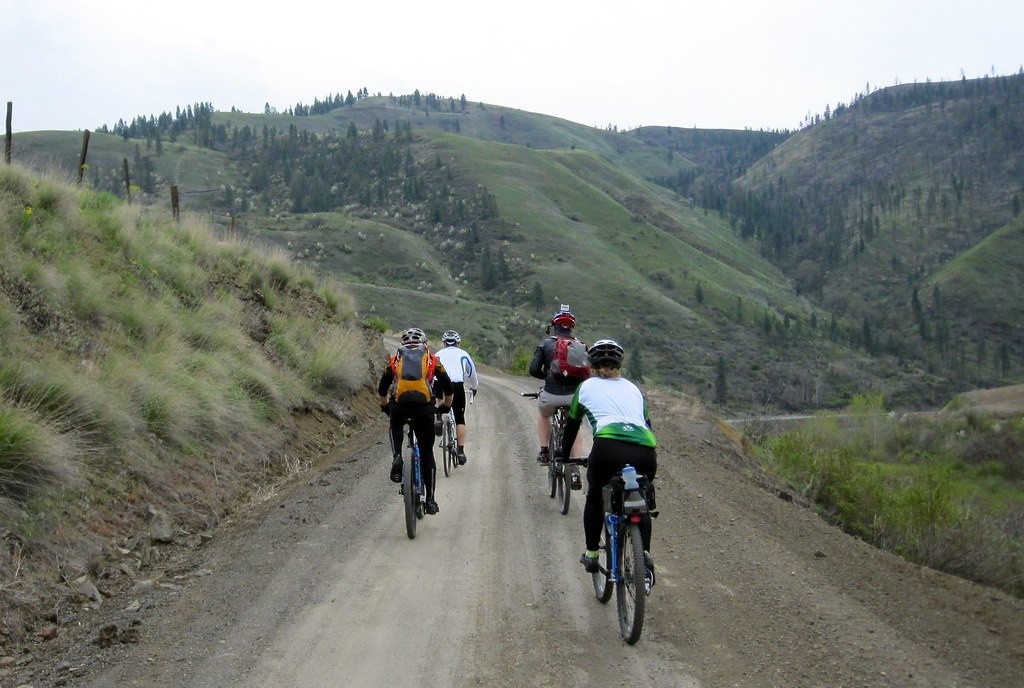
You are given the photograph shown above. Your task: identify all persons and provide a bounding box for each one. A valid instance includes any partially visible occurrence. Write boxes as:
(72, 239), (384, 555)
(555, 340), (659, 586)
(529, 311), (590, 490)
(433, 330), (478, 465)
(378, 328), (453, 515)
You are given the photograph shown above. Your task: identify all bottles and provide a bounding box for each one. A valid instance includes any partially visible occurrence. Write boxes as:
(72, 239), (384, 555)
(605, 511), (612, 535)
(622, 464), (639, 490)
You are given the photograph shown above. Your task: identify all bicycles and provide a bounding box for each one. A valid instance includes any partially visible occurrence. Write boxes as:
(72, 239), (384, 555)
(383, 401), (450, 540)
(430, 388), (474, 477)
(520, 389), (575, 514)
(555, 454), (655, 646)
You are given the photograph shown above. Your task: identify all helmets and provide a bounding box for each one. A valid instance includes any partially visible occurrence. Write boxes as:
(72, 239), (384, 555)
(442, 329), (461, 343)
(552, 311), (577, 327)
(400, 327), (429, 345)
(588, 340), (625, 365)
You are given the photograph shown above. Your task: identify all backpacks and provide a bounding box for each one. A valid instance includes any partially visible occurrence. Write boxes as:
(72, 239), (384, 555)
(550, 334), (590, 381)
(396, 343), (433, 408)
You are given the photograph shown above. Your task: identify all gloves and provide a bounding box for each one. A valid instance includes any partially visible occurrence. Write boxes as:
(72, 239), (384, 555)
(469, 388), (477, 398)
(381, 404), (390, 414)
(437, 404), (452, 415)
(554, 447), (565, 460)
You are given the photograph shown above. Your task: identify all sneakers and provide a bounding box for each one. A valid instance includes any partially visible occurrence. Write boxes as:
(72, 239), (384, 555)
(570, 473), (583, 490)
(537, 452), (551, 463)
(433, 420), (442, 438)
(426, 501), (439, 514)
(580, 552), (599, 574)
(457, 454), (467, 465)
(390, 454), (404, 484)
(641, 555), (655, 585)
